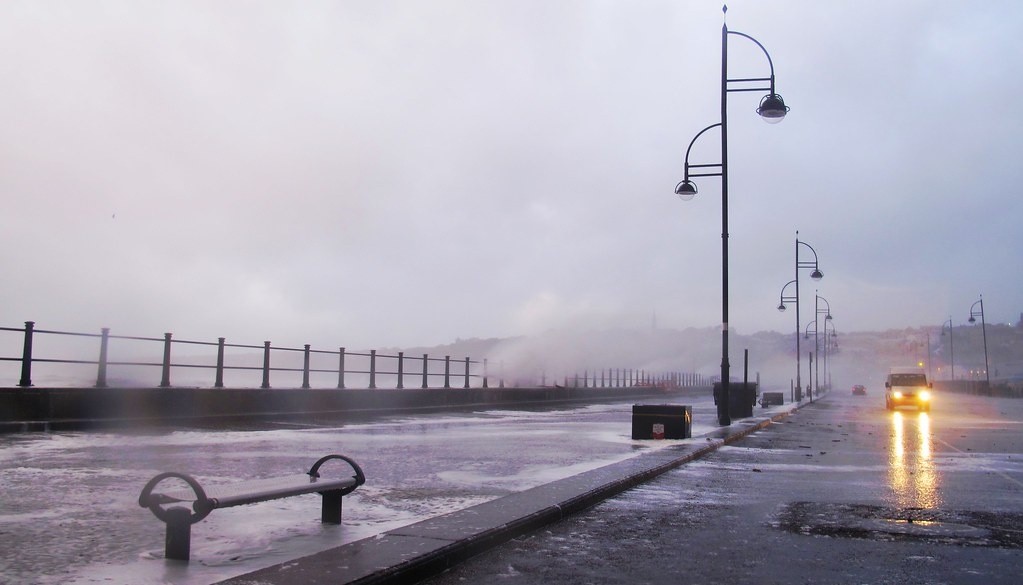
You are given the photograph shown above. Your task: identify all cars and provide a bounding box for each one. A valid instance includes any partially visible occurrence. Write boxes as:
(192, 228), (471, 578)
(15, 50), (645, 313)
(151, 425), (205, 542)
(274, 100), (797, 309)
(852, 384), (866, 395)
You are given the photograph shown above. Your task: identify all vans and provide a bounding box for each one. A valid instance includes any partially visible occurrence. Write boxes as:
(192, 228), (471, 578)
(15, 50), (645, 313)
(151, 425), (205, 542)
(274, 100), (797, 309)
(886, 367), (933, 412)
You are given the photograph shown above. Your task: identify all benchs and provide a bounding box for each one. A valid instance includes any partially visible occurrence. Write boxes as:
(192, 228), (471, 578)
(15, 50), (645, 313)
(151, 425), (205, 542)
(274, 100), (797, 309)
(138, 453), (365, 561)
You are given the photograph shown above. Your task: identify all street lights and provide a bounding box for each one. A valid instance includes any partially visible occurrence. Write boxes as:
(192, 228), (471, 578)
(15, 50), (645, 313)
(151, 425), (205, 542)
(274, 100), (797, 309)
(819, 315), (838, 393)
(804, 289), (834, 397)
(776, 229), (824, 403)
(673, 3), (790, 428)
(940, 315), (955, 393)
(968, 294), (991, 397)
(909, 333), (932, 388)
